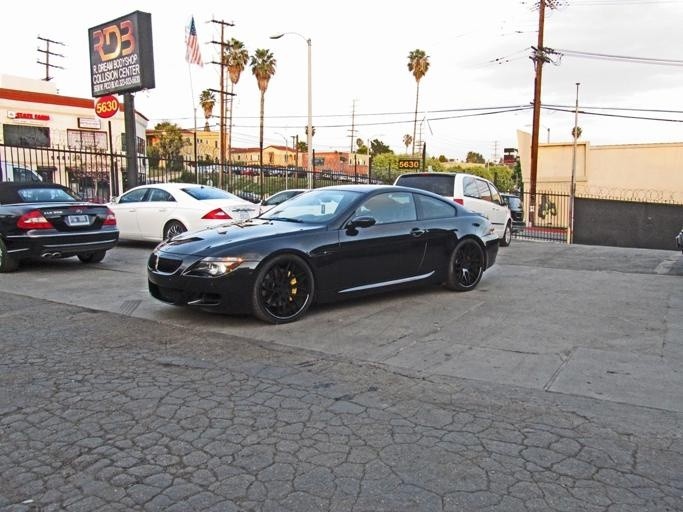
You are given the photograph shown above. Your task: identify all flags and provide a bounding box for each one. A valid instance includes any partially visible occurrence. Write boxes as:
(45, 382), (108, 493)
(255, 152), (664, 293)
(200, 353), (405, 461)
(185, 17), (205, 68)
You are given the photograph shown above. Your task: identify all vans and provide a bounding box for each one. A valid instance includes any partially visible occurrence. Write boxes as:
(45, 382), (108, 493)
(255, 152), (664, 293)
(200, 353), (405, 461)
(392, 171), (516, 248)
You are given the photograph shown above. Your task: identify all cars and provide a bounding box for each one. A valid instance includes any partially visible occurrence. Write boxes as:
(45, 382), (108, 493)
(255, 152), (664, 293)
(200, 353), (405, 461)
(146, 182), (502, 325)
(0, 180), (120, 274)
(93, 180), (261, 247)
(248, 184), (315, 218)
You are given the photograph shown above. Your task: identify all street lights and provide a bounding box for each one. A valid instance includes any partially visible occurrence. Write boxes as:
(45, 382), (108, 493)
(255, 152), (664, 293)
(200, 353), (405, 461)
(267, 28), (320, 191)
